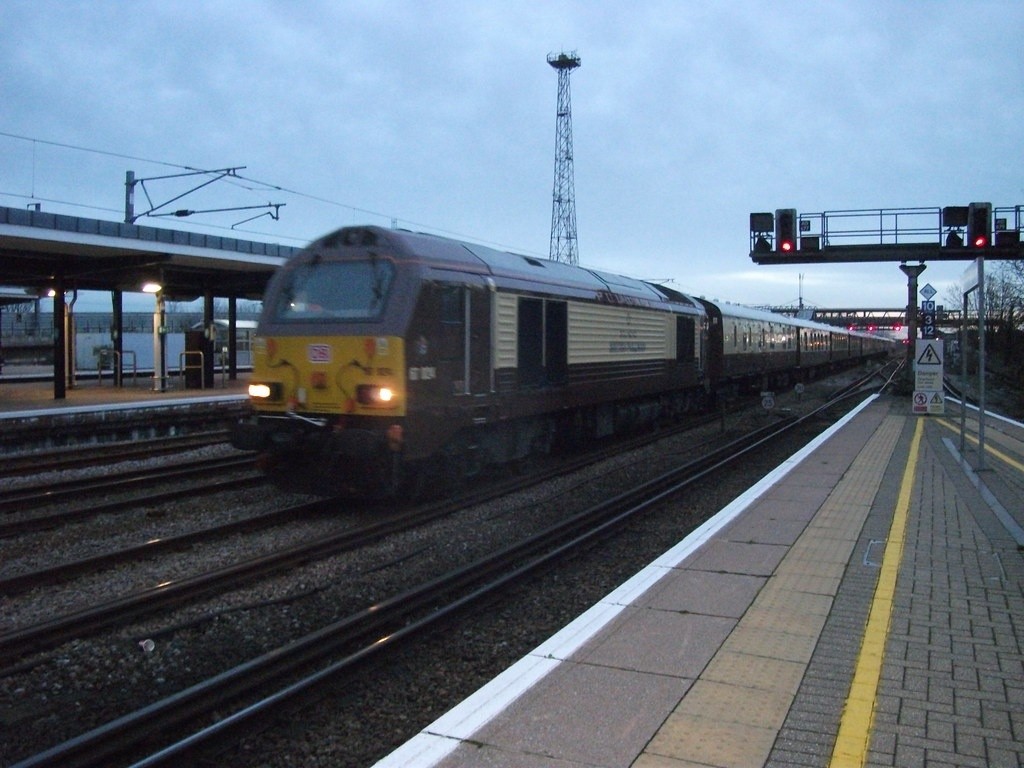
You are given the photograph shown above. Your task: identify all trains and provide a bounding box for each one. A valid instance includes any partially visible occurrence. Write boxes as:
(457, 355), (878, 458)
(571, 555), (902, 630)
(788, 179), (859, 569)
(230, 222), (901, 487)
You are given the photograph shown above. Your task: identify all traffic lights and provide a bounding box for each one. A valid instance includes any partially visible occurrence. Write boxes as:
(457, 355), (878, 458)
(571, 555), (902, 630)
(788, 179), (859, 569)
(775, 208), (797, 252)
(969, 203), (991, 248)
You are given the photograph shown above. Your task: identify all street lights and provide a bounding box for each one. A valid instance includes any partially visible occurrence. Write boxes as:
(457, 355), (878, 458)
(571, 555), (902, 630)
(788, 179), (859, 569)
(140, 282), (164, 390)
(47, 289), (75, 388)
